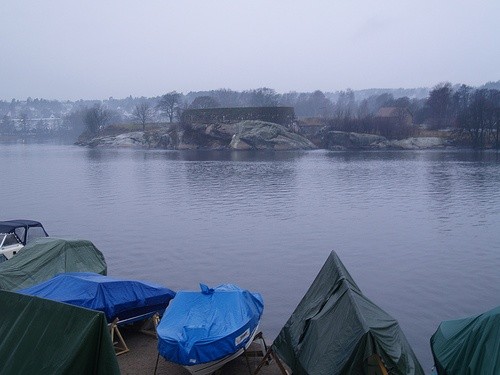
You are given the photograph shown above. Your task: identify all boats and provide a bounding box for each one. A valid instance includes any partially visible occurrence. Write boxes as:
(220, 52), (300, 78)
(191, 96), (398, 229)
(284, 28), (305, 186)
(157, 283), (265, 375)
